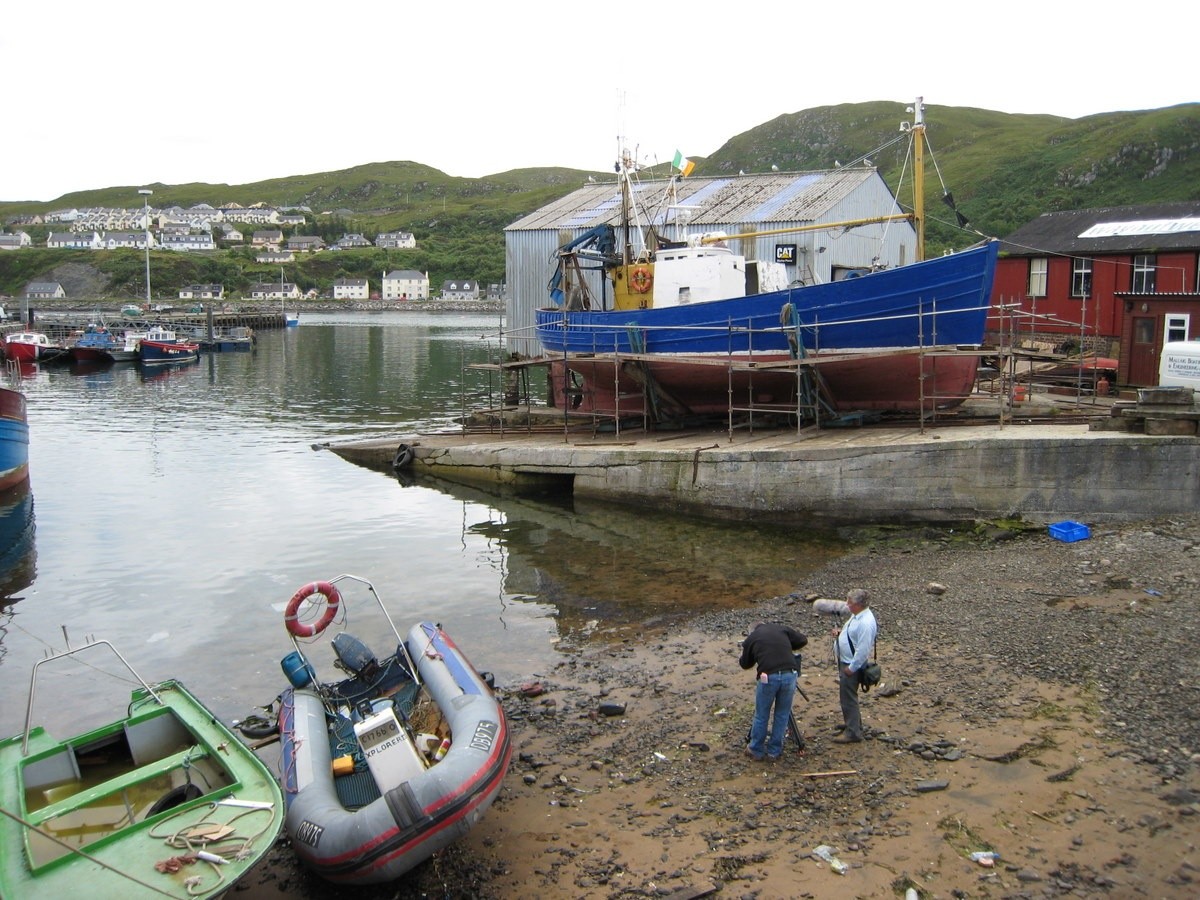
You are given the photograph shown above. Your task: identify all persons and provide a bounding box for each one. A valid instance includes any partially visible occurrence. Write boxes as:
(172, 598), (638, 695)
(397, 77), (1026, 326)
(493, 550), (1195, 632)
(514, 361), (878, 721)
(830, 588), (878, 744)
(738, 620), (808, 762)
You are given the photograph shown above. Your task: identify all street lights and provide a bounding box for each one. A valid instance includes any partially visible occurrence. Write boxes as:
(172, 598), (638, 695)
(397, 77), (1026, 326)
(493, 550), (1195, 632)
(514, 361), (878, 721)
(138, 189), (154, 312)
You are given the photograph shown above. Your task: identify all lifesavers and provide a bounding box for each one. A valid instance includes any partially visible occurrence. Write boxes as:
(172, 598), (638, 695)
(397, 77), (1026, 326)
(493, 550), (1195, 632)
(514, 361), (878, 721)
(284, 581), (339, 637)
(630, 266), (652, 294)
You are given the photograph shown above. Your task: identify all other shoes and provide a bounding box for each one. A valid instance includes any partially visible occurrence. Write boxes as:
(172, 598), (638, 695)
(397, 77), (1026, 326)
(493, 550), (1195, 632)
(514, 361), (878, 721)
(833, 733), (860, 743)
(767, 754), (777, 762)
(835, 723), (845, 729)
(743, 748), (763, 761)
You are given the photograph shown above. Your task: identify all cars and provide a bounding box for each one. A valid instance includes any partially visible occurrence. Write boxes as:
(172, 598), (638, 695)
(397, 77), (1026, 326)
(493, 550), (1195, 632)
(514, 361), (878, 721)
(120, 305), (144, 317)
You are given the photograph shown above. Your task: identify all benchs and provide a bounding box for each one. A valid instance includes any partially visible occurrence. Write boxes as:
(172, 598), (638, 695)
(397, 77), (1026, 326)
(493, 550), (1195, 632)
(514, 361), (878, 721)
(28, 744), (209, 831)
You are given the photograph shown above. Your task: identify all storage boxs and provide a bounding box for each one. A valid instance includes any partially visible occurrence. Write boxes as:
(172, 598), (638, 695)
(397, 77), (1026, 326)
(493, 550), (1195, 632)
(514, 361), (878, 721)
(1048, 520), (1090, 543)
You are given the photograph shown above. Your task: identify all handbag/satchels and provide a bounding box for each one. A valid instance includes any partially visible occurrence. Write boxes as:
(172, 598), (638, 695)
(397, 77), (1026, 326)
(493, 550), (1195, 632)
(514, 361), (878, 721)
(856, 664), (881, 692)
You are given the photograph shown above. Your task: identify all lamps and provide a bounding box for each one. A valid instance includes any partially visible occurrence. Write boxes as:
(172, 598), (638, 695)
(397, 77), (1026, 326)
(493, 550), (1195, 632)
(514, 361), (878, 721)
(1141, 303), (1148, 313)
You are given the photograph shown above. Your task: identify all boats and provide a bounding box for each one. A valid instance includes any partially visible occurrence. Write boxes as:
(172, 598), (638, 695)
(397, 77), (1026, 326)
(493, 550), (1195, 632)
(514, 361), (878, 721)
(138, 340), (200, 368)
(278, 572), (512, 888)
(0, 388), (31, 492)
(0, 625), (285, 900)
(0, 477), (37, 601)
(532, 95), (1001, 421)
(69, 332), (137, 365)
(241, 716), (278, 743)
(5, 331), (69, 364)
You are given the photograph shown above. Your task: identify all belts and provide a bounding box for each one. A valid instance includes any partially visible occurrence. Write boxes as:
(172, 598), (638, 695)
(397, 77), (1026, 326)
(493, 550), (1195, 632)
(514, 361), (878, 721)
(777, 669), (796, 673)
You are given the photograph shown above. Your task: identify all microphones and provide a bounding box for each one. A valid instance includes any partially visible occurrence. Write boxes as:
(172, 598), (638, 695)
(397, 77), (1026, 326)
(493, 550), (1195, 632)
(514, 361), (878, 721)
(812, 599), (850, 616)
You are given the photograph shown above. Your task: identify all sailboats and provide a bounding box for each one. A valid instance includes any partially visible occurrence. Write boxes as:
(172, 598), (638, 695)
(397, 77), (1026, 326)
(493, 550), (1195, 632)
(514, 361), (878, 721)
(280, 267), (299, 327)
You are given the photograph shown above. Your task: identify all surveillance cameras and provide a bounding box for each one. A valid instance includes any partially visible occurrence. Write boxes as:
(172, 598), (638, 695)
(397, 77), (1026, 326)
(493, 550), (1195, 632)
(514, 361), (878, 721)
(905, 109), (910, 114)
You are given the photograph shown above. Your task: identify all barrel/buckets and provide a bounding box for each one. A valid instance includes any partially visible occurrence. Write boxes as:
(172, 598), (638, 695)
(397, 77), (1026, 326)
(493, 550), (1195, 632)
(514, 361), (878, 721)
(280, 652), (315, 688)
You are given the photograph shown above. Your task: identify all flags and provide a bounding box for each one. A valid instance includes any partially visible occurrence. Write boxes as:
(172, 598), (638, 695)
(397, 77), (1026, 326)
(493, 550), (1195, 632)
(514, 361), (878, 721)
(671, 149), (695, 178)
